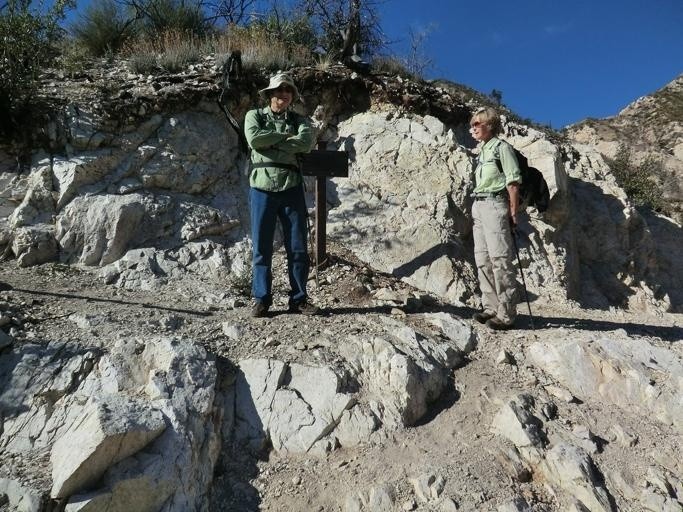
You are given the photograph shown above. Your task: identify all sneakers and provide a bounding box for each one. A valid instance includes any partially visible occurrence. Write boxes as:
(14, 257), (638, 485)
(472, 312), (495, 324)
(290, 302), (321, 315)
(486, 317), (513, 329)
(251, 303), (269, 317)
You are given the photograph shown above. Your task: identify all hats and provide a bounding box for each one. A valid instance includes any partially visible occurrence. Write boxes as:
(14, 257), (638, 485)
(259, 73), (300, 104)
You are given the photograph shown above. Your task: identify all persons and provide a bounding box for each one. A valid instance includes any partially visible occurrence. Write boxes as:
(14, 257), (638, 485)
(470, 108), (522, 333)
(243, 72), (319, 317)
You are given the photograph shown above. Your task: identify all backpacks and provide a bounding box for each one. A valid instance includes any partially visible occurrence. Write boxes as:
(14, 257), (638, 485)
(494, 139), (530, 206)
